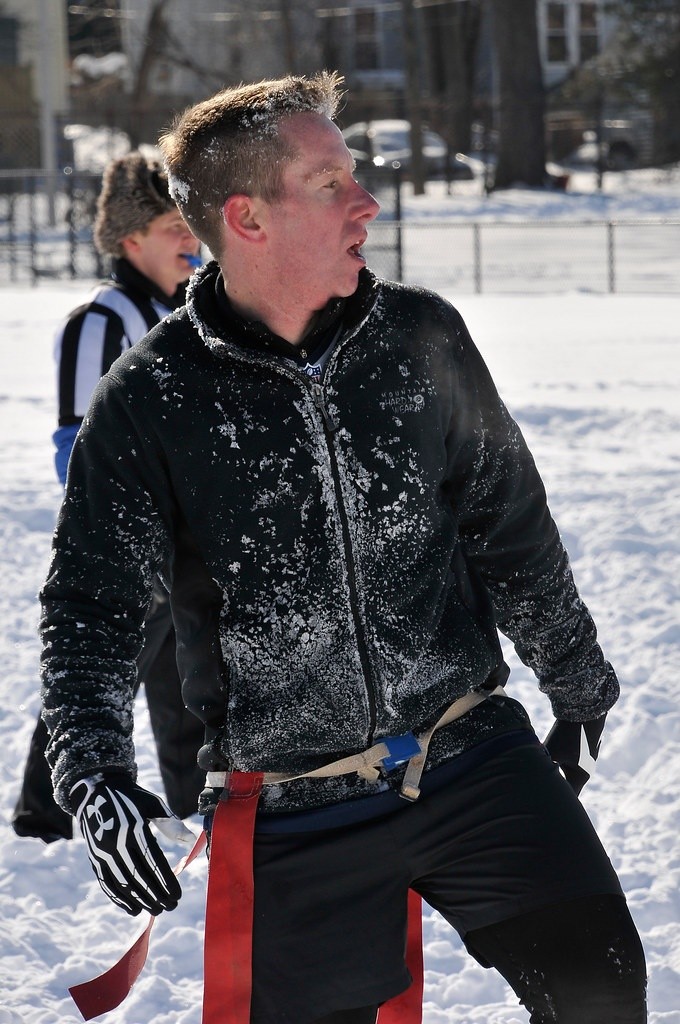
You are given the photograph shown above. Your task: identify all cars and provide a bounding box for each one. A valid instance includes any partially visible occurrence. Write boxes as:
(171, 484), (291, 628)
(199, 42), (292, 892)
(339, 117), (475, 185)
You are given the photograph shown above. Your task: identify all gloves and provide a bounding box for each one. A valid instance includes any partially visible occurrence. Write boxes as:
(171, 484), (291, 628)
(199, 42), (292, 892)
(545, 713), (607, 800)
(69, 764), (198, 917)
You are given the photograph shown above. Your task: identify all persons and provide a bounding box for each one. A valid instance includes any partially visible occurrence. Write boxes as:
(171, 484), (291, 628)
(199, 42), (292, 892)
(39, 71), (650, 1024)
(50, 154), (206, 820)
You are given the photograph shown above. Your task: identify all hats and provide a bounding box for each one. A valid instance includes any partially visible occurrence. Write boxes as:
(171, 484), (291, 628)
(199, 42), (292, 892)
(92, 155), (178, 253)
